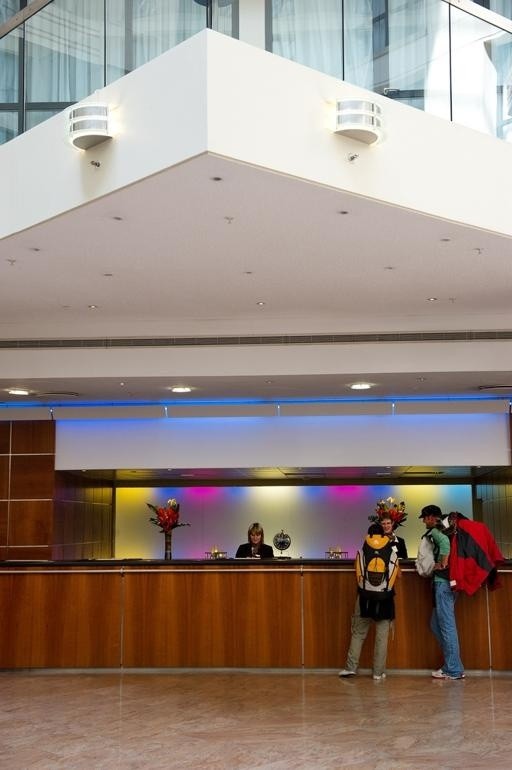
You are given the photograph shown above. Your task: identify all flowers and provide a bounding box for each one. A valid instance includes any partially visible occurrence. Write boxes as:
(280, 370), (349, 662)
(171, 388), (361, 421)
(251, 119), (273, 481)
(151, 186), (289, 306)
(146, 498), (193, 534)
(367, 495), (408, 533)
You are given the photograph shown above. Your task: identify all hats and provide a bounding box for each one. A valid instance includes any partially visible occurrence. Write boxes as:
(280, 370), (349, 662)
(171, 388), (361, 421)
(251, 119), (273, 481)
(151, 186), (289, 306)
(418, 505), (441, 518)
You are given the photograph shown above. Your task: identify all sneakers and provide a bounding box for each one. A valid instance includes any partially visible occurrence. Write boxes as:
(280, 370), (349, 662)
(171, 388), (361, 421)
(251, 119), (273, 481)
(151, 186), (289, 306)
(432, 670), (465, 680)
(373, 674), (386, 679)
(338, 669), (356, 678)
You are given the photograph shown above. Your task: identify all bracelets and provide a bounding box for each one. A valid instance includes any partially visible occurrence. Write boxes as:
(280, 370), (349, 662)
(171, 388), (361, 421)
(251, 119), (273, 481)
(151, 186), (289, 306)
(441, 564), (449, 570)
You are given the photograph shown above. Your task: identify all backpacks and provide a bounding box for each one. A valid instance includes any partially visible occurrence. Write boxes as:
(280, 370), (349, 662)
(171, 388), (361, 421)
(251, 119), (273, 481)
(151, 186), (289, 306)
(415, 526), (440, 577)
(360, 540), (394, 593)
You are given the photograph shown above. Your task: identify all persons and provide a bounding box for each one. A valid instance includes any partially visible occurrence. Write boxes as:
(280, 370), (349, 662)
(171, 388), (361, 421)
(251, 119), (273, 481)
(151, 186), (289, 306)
(234, 522), (275, 558)
(378, 512), (408, 559)
(415, 504), (467, 681)
(338, 524), (401, 682)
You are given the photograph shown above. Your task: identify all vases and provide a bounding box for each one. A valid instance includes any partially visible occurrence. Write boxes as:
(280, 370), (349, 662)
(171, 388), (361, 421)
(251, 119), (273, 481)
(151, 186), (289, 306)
(164, 532), (172, 561)
